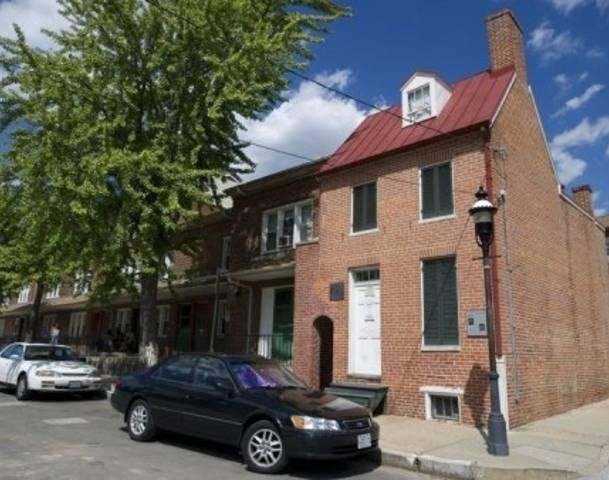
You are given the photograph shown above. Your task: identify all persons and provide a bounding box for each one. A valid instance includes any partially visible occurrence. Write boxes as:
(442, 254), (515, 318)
(96, 323), (136, 352)
(50, 323), (60, 344)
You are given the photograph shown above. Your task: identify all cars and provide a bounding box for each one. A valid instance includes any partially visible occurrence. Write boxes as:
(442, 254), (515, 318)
(106, 349), (381, 475)
(0, 341), (103, 399)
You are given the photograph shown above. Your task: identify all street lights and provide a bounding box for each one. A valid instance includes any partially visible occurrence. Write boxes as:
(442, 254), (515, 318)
(463, 186), (511, 457)
